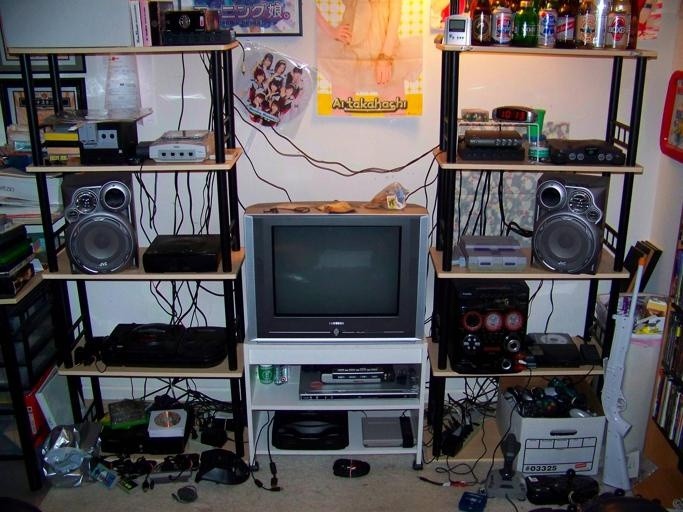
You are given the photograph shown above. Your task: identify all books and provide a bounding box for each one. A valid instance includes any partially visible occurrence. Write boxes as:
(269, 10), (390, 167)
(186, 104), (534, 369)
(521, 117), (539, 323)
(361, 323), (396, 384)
(35, 363), (74, 432)
(650, 236), (683, 454)
(0, 222), (36, 299)
(43, 125), (81, 161)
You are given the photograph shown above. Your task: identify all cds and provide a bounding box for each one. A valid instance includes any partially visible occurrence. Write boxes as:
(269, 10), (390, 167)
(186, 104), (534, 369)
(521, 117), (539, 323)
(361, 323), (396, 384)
(541, 334), (568, 347)
(154, 410), (181, 429)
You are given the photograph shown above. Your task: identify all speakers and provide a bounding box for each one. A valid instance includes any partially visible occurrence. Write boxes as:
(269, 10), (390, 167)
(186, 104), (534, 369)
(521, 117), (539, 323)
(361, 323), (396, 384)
(447, 278), (529, 374)
(60, 172), (139, 273)
(531, 173), (608, 275)
(444, 15), (472, 47)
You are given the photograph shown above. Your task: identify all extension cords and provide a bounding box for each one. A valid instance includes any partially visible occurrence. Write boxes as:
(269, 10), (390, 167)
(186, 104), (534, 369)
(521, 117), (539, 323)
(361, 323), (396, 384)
(445, 416), (481, 456)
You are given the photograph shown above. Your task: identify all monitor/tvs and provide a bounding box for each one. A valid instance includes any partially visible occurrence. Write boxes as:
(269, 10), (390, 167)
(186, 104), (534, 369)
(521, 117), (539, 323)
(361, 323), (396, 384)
(242, 202), (428, 344)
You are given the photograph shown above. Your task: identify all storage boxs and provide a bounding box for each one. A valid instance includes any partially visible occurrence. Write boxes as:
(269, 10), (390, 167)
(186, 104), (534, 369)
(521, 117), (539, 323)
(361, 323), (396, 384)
(492, 376), (607, 478)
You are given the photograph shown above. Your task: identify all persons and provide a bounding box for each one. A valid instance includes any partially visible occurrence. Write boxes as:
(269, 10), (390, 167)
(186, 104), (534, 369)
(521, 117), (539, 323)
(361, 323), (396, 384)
(319, 0), (405, 116)
(242, 53), (303, 129)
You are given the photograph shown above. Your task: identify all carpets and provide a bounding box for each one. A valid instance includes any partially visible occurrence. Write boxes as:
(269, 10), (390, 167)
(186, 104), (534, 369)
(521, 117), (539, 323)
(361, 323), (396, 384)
(34, 408), (638, 512)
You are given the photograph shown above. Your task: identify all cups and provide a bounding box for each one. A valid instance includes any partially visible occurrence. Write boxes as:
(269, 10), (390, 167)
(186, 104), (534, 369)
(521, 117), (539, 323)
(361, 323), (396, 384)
(528, 110), (545, 136)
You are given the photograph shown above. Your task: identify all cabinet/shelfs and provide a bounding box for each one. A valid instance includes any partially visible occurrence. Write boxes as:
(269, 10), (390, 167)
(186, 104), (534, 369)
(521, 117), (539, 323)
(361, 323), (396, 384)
(649, 204), (683, 472)
(239, 343), (431, 468)
(426, 32), (655, 468)
(10, 38), (248, 462)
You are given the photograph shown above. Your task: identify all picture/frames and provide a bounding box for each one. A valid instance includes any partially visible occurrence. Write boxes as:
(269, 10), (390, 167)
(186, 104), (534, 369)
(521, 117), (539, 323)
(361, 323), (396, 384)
(1, 76), (89, 147)
(0, 27), (88, 73)
(658, 68), (682, 165)
(175, 0), (303, 36)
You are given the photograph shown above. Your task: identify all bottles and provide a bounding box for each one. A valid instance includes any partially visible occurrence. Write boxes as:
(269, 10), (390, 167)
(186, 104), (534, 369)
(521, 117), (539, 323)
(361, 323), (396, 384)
(576, 0), (594, 49)
(605, 0), (631, 50)
(557, 1), (577, 48)
(628, 0), (637, 50)
(522, 134), (549, 164)
(257, 365), (287, 385)
(513, 1), (539, 46)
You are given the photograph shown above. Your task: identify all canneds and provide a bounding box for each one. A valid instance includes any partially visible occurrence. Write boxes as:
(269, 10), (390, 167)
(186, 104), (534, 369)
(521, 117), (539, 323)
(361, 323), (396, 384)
(605, 11), (632, 50)
(537, 6), (556, 48)
(492, 6), (514, 47)
(258, 364), (286, 386)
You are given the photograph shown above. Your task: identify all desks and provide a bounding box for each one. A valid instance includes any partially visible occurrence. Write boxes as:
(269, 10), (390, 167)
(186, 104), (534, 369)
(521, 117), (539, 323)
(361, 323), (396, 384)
(1, 257), (87, 496)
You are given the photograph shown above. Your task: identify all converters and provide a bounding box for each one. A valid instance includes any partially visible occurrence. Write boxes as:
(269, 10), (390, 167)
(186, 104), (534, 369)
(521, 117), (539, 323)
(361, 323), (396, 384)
(202, 429), (226, 448)
(444, 434), (462, 455)
